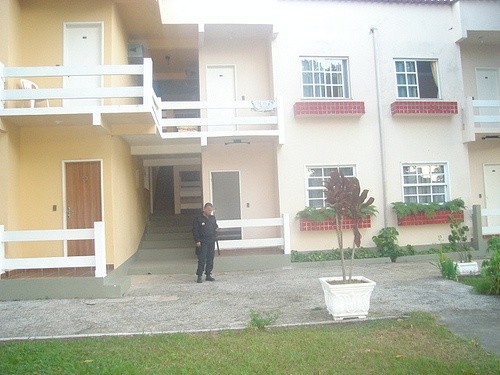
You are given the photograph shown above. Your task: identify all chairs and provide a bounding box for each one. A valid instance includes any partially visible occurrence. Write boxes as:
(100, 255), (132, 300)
(20, 79), (48, 108)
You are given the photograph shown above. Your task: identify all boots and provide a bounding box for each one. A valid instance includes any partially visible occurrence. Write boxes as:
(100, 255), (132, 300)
(205, 273), (215, 281)
(197, 275), (202, 282)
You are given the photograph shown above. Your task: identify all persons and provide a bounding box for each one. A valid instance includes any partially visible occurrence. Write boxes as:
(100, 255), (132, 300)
(192, 203), (219, 283)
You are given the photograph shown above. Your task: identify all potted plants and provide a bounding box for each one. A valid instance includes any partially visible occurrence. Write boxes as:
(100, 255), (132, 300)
(319, 169), (376, 322)
(449, 212), (478, 276)
